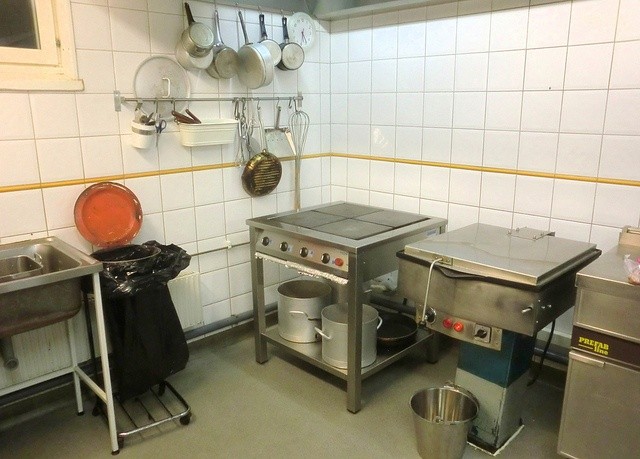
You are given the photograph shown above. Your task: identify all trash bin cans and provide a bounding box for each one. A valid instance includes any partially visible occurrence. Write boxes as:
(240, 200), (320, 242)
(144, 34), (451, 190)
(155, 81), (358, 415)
(77, 180), (193, 399)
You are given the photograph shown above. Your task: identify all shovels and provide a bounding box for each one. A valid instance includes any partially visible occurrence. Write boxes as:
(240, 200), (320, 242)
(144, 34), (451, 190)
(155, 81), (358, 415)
(265, 105), (297, 159)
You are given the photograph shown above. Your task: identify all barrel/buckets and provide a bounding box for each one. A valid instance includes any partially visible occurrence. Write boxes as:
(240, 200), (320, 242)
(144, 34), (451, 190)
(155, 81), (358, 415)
(409, 381), (481, 459)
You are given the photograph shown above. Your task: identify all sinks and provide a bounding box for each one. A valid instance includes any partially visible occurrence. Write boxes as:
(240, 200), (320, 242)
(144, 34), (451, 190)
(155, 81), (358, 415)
(0, 234), (103, 339)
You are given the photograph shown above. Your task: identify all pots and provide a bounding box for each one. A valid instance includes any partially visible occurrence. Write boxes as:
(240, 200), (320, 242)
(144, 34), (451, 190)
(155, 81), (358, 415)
(182, 2), (214, 56)
(278, 15), (306, 71)
(176, 22), (214, 72)
(259, 12), (283, 68)
(207, 8), (238, 79)
(242, 117), (281, 198)
(374, 313), (418, 348)
(238, 11), (274, 91)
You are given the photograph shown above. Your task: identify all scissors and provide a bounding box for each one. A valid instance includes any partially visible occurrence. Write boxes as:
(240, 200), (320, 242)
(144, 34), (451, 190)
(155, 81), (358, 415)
(154, 119), (168, 147)
